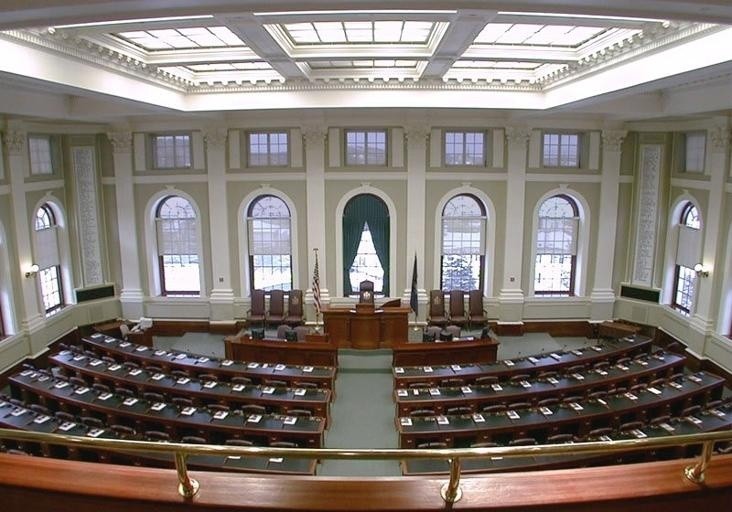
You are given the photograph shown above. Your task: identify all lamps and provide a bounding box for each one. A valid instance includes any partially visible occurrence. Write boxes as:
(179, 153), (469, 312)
(694, 262), (711, 278)
(26, 261), (39, 279)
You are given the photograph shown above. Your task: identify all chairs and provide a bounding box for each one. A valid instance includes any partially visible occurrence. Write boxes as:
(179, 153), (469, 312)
(426, 289), (489, 330)
(236, 288), (305, 328)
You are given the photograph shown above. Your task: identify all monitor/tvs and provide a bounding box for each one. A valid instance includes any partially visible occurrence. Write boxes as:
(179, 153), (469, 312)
(480, 327), (489, 340)
(285, 330), (297, 341)
(252, 328), (265, 339)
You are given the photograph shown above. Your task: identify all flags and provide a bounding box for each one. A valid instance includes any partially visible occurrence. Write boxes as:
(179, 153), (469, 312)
(409, 255), (420, 317)
(311, 248), (323, 316)
